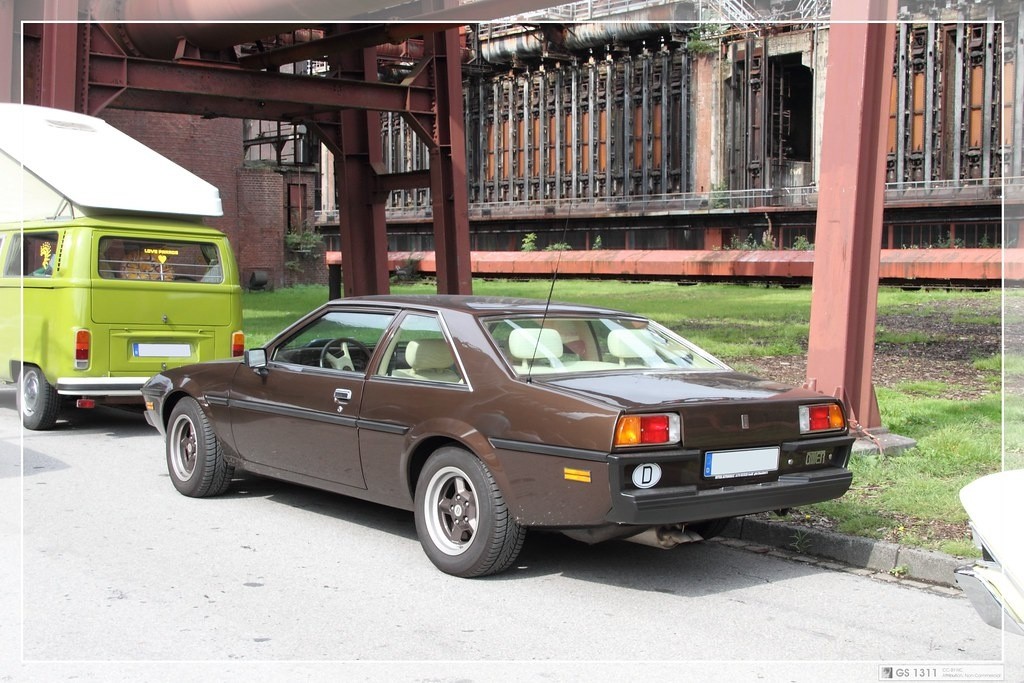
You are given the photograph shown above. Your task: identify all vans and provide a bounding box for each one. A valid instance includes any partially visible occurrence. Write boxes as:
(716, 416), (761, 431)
(0, 213), (246, 430)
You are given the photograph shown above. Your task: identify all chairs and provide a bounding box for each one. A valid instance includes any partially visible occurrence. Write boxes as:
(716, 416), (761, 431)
(391, 338), (460, 383)
(509, 328), (564, 374)
(608, 329), (655, 368)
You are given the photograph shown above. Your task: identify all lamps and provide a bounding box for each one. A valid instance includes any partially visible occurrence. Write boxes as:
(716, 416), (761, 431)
(244, 270), (268, 292)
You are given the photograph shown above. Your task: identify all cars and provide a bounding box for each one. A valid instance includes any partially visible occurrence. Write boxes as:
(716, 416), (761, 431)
(237, 434), (246, 437)
(144, 297), (853, 580)
